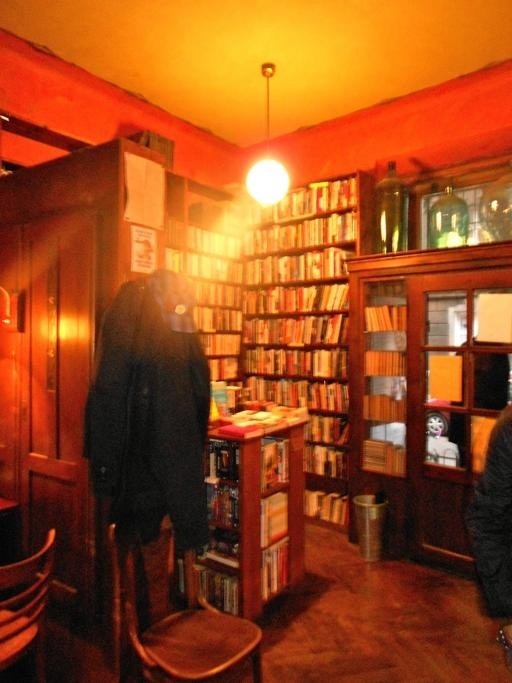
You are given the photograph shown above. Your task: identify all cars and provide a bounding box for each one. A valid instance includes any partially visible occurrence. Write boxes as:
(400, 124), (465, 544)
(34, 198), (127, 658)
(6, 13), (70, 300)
(426, 399), (452, 434)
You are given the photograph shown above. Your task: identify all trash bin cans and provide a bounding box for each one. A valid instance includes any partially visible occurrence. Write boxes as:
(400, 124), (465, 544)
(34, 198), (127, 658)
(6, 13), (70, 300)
(352, 494), (390, 562)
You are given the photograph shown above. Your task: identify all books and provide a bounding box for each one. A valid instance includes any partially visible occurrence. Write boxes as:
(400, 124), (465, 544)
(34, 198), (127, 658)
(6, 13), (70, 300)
(167, 175), (359, 616)
(363, 300), (406, 477)
